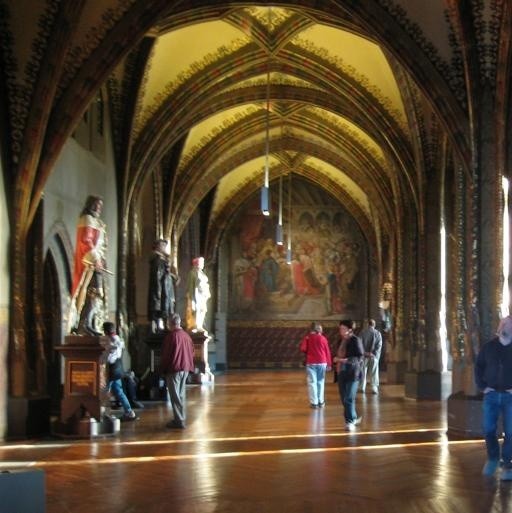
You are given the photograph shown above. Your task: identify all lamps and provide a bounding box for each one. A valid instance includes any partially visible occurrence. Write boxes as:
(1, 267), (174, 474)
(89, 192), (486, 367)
(260, 7), (295, 265)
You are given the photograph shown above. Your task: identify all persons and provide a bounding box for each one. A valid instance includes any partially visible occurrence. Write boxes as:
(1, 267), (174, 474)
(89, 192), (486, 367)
(291, 254), (319, 295)
(298, 319), (333, 409)
(121, 374), (146, 408)
(357, 318), (385, 394)
(257, 247), (281, 292)
(332, 261), (355, 315)
(184, 256), (211, 335)
(147, 237), (179, 334)
(68, 192), (111, 337)
(314, 262), (338, 314)
(99, 320), (137, 422)
(243, 254), (258, 301)
(330, 319), (367, 432)
(157, 312), (195, 430)
(472, 314), (511, 483)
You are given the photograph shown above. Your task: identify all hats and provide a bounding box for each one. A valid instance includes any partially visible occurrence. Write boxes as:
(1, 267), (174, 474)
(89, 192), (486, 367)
(498, 317), (512, 346)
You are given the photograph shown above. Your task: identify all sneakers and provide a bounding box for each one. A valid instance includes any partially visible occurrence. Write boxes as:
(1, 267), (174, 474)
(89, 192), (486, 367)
(311, 404), (319, 410)
(501, 469), (512, 481)
(482, 458), (498, 476)
(320, 402), (326, 408)
(166, 419), (185, 429)
(360, 386), (379, 394)
(344, 419), (357, 431)
(118, 412), (136, 422)
